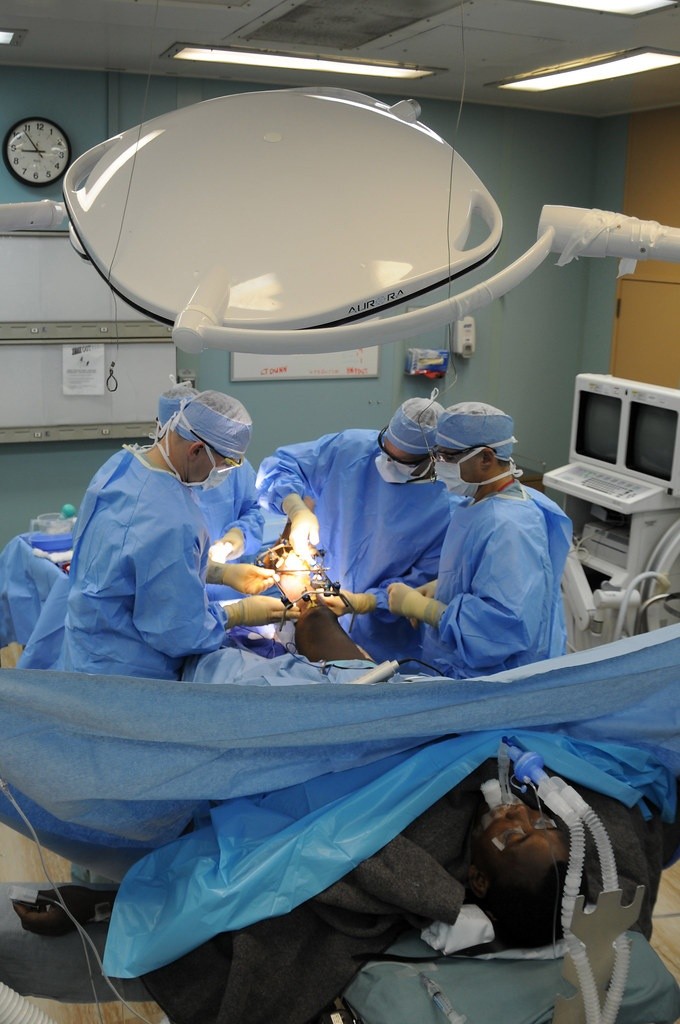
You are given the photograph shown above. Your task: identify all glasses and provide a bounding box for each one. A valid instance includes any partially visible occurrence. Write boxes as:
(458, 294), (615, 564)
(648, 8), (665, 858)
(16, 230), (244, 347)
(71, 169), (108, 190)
(376, 426), (429, 466)
(189, 429), (244, 474)
(430, 442), (497, 464)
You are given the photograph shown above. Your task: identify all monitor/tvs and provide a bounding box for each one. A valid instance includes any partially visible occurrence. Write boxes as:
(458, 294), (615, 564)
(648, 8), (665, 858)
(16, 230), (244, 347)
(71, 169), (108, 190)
(569, 375), (680, 496)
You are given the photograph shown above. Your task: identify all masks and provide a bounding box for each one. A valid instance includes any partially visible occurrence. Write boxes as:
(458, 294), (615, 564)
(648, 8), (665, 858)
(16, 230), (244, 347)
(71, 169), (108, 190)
(189, 445), (231, 492)
(374, 451), (433, 483)
(434, 447), (487, 497)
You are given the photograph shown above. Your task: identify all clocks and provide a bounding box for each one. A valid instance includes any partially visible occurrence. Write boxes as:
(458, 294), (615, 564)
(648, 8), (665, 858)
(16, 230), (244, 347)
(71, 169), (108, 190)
(2, 116), (72, 187)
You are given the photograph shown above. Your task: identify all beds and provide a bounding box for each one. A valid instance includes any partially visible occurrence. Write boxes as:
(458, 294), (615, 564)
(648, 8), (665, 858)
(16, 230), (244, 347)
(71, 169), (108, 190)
(341, 923), (680, 1024)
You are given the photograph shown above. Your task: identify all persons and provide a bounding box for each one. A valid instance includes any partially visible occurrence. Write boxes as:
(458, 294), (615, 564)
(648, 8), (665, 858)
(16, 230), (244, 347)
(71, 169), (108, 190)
(386, 403), (573, 679)
(61, 389), (301, 680)
(255, 396), (451, 674)
(278, 549), (588, 948)
(156, 378), (266, 601)
